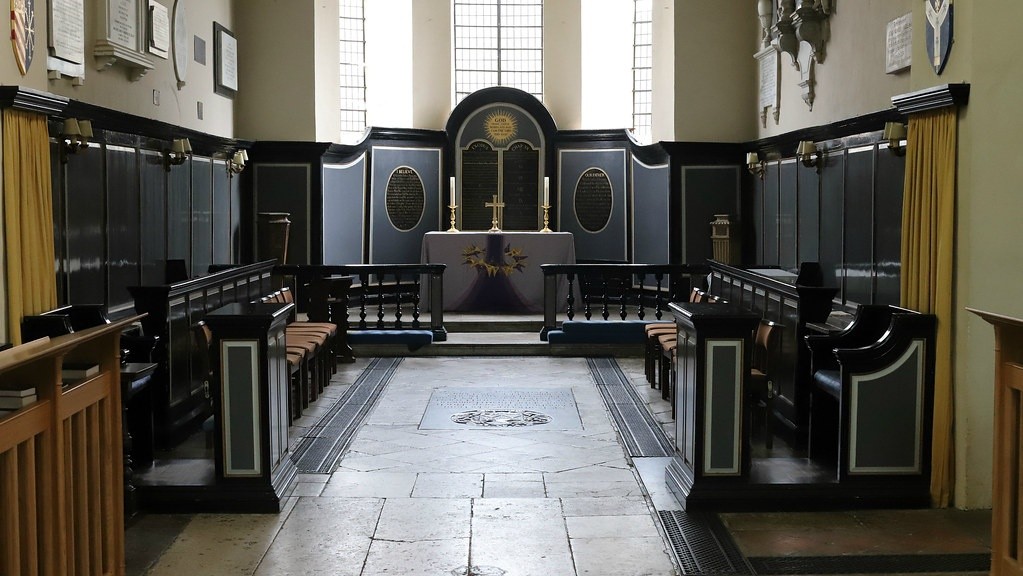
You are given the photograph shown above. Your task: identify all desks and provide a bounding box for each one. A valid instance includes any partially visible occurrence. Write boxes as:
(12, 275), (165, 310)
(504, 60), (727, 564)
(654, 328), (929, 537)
(419, 231), (582, 313)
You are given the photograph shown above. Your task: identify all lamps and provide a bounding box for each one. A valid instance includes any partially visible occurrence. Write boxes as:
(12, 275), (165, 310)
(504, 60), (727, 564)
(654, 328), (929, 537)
(797, 141), (823, 175)
(163, 137), (192, 172)
(883, 120), (907, 149)
(747, 152), (764, 179)
(59, 118), (94, 163)
(230, 148), (249, 178)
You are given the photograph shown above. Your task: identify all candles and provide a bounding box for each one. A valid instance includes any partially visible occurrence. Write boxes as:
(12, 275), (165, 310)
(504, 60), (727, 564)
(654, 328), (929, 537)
(544, 177), (549, 207)
(450, 177), (455, 206)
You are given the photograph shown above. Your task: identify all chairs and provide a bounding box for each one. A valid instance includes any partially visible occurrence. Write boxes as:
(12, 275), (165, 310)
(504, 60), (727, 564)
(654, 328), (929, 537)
(645, 286), (786, 451)
(191, 287), (338, 427)
